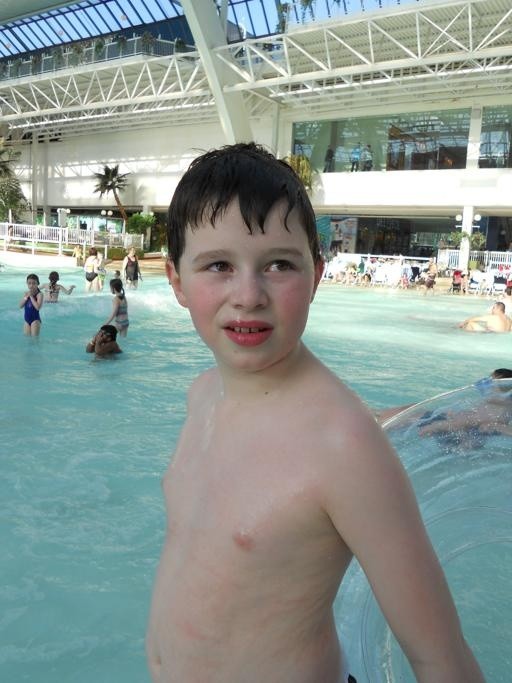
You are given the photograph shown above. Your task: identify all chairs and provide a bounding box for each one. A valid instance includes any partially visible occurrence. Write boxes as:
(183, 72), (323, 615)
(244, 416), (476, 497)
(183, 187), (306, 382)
(327, 258), (507, 296)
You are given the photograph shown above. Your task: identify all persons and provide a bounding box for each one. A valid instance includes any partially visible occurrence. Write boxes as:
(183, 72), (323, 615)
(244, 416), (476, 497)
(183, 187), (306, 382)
(19, 242), (142, 359)
(143, 139), (486, 683)
(377, 370), (512, 454)
(349, 141), (373, 172)
(341, 253), (512, 332)
(323, 145), (334, 172)
